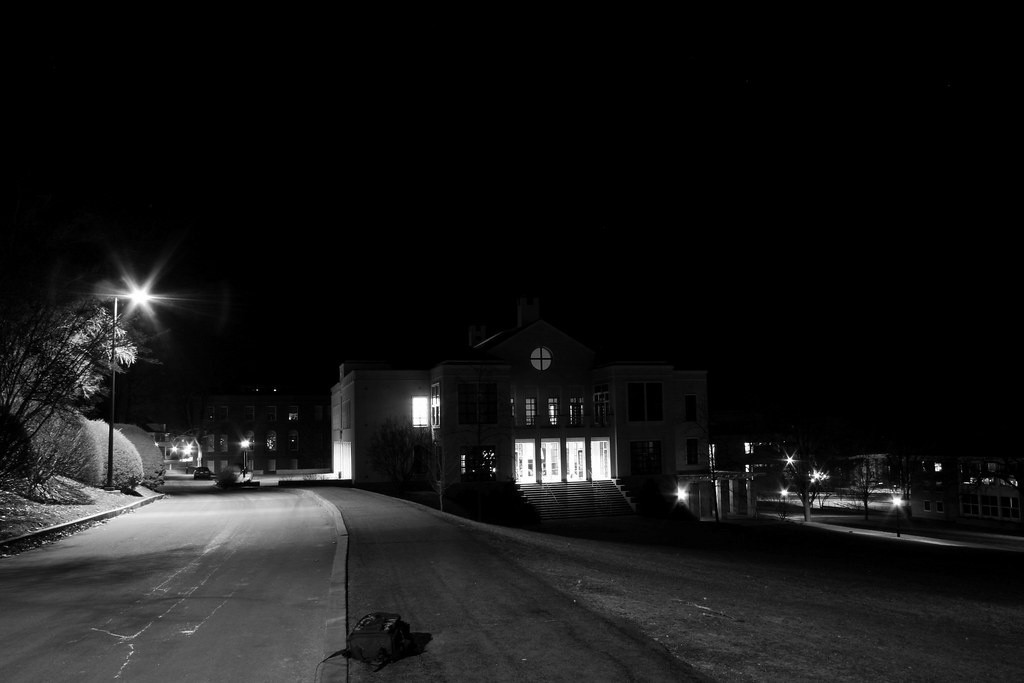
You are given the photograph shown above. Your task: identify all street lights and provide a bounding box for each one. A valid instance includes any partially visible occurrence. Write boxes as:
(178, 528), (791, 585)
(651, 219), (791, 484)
(243, 441), (250, 478)
(104, 289), (150, 491)
(893, 497), (902, 537)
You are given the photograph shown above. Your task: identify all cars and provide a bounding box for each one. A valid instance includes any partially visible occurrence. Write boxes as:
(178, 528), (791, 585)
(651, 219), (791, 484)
(193, 466), (212, 480)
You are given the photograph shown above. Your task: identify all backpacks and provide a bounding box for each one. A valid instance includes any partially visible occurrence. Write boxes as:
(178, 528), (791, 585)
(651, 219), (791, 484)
(342, 611), (411, 672)
